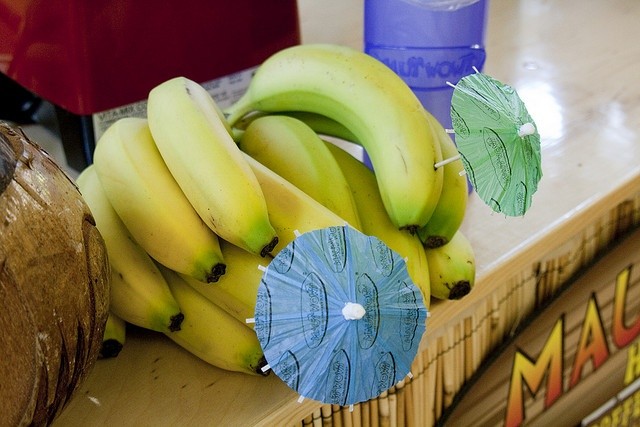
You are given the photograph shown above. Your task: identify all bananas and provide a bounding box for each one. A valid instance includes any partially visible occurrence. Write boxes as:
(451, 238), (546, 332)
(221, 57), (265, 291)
(76, 45), (475, 376)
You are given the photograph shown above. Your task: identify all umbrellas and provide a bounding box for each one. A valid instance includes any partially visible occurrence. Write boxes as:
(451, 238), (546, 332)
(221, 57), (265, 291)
(434, 66), (543, 221)
(246, 221), (431, 412)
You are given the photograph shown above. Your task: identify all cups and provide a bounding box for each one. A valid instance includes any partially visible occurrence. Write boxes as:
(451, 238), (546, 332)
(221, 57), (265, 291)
(364, 1), (485, 194)
